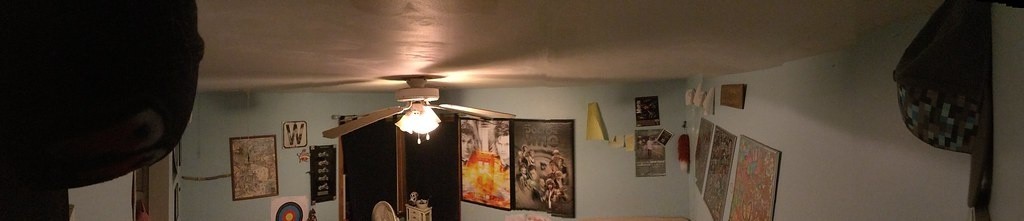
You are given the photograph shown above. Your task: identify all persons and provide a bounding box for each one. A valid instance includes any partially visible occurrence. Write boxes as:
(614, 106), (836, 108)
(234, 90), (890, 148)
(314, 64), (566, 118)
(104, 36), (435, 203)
(518, 146), (567, 209)
(495, 124), (509, 173)
(647, 137), (658, 157)
(461, 121), (475, 168)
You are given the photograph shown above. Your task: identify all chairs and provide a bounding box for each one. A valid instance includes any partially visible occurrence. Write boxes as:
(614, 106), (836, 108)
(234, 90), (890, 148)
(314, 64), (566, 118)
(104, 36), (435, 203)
(372, 200), (399, 221)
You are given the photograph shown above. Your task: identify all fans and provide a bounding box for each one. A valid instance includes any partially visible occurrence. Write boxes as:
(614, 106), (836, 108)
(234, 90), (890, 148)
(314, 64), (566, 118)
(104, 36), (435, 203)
(322, 77), (515, 139)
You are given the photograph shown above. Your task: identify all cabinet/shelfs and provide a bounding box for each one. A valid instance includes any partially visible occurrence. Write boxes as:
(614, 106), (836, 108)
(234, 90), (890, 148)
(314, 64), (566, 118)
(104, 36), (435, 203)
(405, 203), (432, 221)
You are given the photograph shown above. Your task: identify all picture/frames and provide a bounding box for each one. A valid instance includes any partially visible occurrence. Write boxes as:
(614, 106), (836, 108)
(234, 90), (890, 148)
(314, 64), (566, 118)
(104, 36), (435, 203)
(229, 136), (279, 200)
(458, 116), (515, 212)
(514, 118), (575, 217)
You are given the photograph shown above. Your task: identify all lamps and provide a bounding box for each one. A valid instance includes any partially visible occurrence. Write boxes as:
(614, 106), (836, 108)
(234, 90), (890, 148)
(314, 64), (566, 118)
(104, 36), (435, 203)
(395, 100), (441, 144)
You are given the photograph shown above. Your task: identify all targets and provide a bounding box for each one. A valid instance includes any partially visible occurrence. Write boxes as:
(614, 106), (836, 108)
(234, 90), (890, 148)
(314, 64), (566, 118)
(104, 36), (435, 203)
(271, 194), (310, 221)
